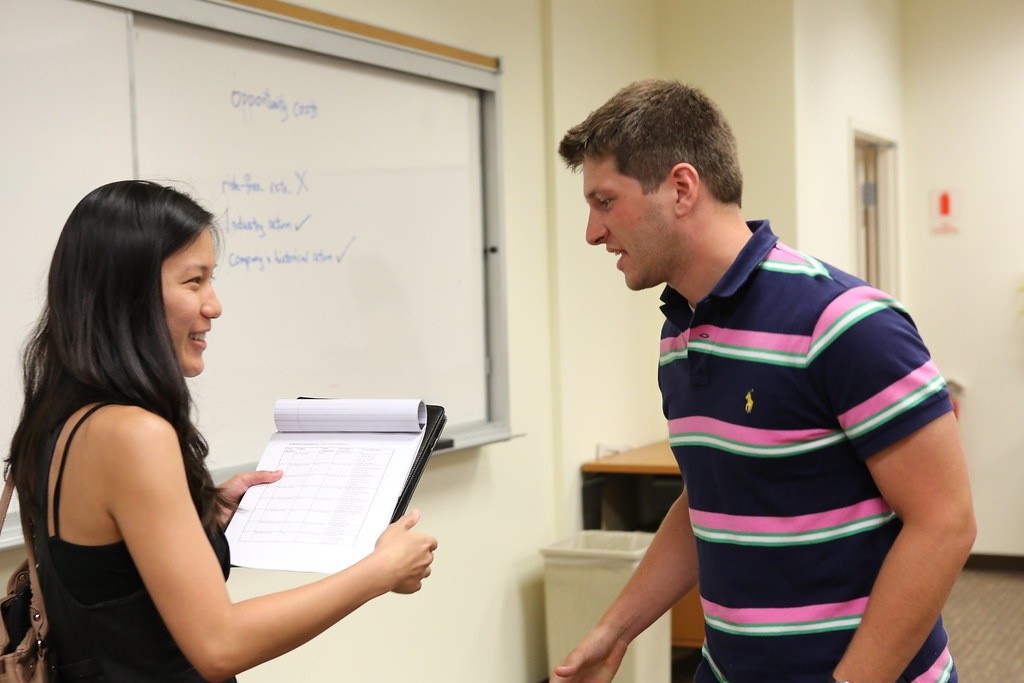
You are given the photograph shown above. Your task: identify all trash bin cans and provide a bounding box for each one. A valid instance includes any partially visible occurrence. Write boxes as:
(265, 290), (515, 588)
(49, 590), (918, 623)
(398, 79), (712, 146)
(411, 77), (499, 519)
(540, 529), (674, 683)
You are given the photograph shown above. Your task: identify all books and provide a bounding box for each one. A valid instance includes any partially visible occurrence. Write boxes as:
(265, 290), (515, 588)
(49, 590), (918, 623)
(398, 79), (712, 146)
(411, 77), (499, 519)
(222, 399), (429, 577)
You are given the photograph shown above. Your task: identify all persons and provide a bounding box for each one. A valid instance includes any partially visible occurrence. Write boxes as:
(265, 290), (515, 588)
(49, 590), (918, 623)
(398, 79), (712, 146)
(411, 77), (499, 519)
(556, 78), (976, 683)
(13, 181), (437, 681)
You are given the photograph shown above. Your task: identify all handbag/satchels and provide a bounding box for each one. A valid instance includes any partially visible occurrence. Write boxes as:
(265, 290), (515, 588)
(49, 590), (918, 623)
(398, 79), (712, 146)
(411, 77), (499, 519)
(0, 556), (54, 683)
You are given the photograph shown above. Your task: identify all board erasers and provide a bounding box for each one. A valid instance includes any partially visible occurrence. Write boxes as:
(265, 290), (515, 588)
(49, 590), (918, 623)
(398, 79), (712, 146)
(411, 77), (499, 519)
(432, 437), (454, 451)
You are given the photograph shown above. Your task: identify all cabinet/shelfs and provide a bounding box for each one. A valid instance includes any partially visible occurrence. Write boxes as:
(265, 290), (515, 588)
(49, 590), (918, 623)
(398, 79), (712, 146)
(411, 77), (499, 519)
(582, 439), (706, 649)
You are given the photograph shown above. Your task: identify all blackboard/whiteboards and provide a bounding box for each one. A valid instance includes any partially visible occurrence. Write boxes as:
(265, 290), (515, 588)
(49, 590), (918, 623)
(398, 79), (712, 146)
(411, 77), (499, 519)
(3, 0), (519, 552)
